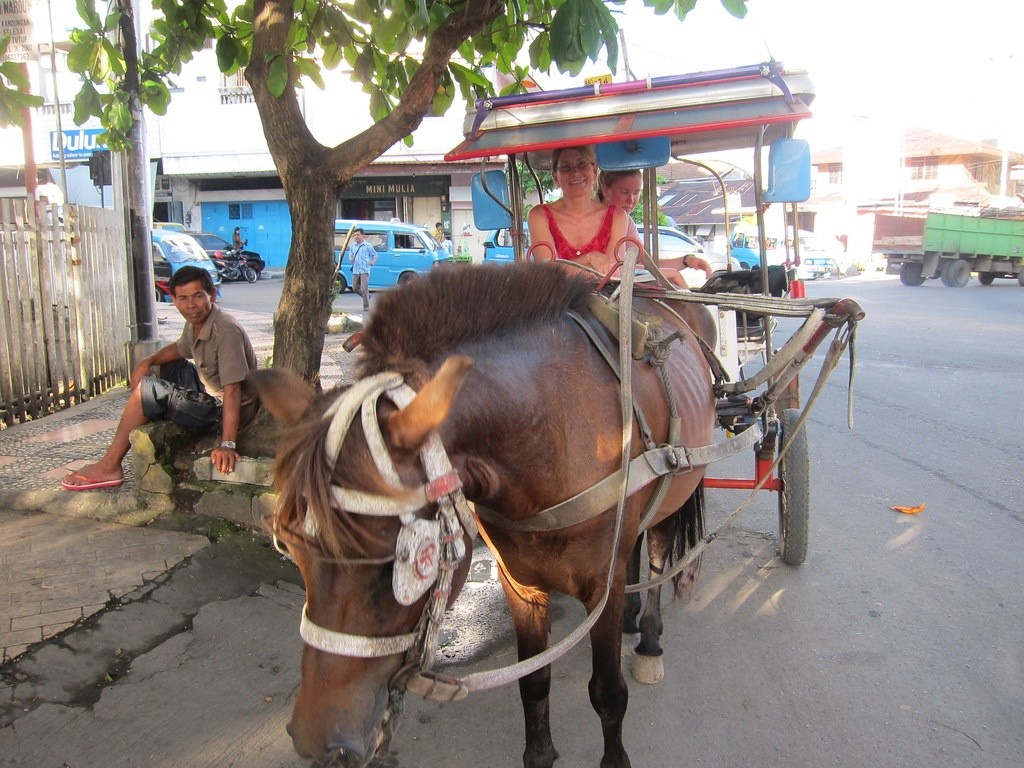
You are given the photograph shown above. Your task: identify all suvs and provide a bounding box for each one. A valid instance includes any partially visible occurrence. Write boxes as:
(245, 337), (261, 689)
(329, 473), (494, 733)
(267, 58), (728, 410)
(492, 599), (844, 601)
(178, 230), (266, 279)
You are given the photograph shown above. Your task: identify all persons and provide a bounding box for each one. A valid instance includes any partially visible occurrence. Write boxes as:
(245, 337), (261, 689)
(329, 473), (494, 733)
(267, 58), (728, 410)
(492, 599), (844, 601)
(349, 228), (378, 311)
(595, 168), (712, 291)
(60, 265), (261, 492)
(233, 227), (242, 249)
(433, 222), (442, 243)
(528, 143), (629, 278)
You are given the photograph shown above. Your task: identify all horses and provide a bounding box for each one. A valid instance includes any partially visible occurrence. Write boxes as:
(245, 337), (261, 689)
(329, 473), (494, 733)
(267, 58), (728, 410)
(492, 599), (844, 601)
(243, 260), (716, 768)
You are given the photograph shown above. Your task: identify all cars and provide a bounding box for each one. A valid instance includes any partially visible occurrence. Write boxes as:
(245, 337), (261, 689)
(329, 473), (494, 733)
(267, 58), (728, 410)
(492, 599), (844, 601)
(635, 224), (744, 289)
(152, 241), (173, 301)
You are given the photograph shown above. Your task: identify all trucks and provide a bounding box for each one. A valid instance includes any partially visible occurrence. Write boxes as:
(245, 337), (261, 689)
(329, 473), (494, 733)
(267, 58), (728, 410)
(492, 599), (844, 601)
(870, 208), (1024, 288)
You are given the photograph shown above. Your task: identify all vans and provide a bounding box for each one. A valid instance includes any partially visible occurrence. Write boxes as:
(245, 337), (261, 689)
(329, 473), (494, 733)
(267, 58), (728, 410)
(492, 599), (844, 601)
(728, 223), (834, 281)
(152, 222), (186, 232)
(152, 229), (223, 303)
(333, 218), (452, 294)
(480, 220), (534, 265)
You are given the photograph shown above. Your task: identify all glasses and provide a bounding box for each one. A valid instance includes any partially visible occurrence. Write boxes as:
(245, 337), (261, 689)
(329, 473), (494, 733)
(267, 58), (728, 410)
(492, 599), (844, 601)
(555, 161), (591, 173)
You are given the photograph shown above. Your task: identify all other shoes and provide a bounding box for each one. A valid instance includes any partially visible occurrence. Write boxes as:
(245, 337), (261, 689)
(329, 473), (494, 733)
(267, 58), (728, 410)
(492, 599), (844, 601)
(364, 306), (369, 311)
(369, 294), (372, 299)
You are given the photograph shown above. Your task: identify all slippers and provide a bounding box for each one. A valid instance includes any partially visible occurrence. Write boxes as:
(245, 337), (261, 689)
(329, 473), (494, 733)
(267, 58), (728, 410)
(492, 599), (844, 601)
(60, 467), (125, 490)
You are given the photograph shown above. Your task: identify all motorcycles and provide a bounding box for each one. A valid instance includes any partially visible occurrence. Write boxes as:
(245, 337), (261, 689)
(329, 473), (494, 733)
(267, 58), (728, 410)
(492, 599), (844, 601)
(211, 238), (258, 283)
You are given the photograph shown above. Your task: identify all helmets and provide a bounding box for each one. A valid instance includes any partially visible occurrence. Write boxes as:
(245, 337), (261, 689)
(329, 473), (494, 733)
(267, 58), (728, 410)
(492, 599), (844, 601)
(213, 252), (224, 260)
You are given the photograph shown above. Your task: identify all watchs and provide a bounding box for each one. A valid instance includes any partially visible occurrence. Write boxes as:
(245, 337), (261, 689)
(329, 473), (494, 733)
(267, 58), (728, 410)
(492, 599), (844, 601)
(220, 440), (236, 449)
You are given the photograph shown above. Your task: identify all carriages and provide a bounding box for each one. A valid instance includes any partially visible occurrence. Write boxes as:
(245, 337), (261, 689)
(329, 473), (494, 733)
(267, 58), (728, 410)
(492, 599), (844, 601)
(244, 51), (865, 767)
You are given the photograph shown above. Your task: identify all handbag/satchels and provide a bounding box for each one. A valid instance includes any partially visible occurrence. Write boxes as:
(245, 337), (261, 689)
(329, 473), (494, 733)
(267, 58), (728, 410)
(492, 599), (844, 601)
(349, 266), (353, 273)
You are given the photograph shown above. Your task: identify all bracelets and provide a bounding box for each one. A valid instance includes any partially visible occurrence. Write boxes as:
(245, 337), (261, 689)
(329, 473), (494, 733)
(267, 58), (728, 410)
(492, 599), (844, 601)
(683, 254), (694, 267)
